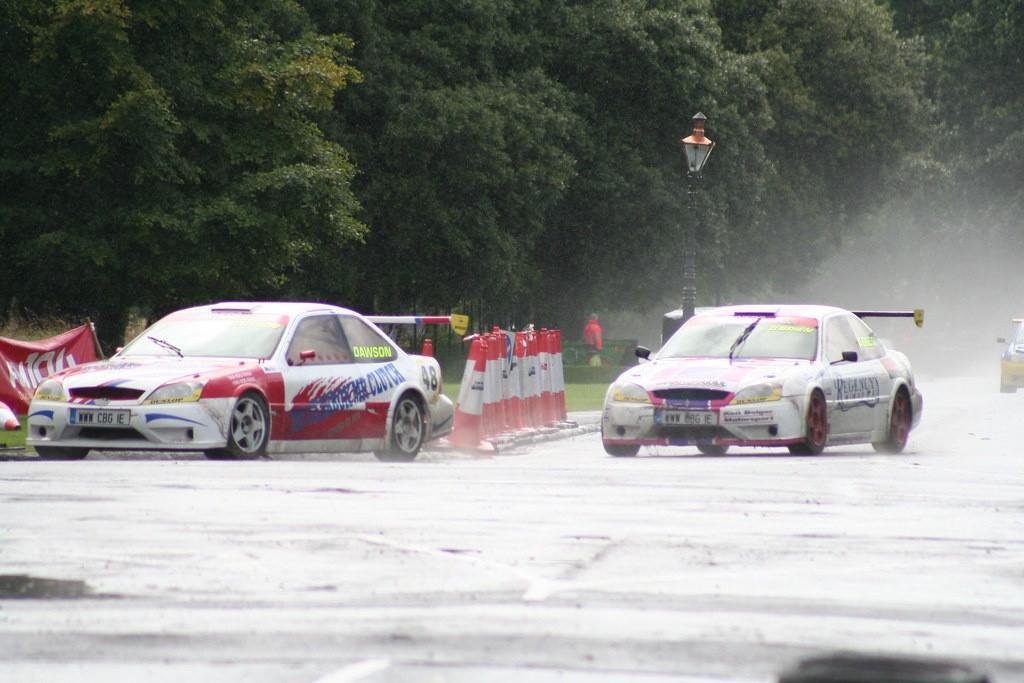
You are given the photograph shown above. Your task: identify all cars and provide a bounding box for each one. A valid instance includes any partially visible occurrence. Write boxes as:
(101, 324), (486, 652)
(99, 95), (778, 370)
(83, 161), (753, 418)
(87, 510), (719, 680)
(600, 302), (923, 455)
(996, 317), (1023, 393)
(27, 301), (473, 458)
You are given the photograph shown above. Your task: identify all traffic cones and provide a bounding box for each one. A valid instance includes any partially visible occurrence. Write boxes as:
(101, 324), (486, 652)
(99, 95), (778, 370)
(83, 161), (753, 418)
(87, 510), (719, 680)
(455, 324), (579, 444)
(1, 400), (24, 434)
(419, 335), (439, 450)
(439, 346), (495, 453)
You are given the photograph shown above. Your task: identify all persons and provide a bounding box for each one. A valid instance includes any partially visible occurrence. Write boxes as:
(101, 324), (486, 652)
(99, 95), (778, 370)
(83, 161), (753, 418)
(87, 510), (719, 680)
(582, 313), (603, 365)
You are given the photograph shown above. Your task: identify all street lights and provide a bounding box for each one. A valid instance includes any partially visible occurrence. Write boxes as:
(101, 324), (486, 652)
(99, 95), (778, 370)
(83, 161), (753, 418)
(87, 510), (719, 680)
(680, 111), (718, 324)
